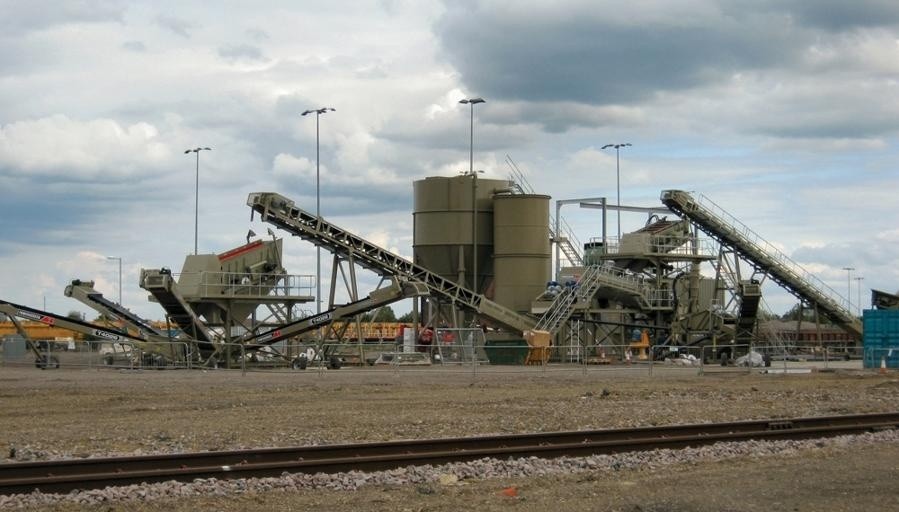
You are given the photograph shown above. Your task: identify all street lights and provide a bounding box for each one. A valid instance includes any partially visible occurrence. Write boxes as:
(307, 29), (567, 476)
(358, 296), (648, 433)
(303, 106), (334, 317)
(601, 142), (630, 241)
(461, 96), (486, 177)
(185, 144), (211, 256)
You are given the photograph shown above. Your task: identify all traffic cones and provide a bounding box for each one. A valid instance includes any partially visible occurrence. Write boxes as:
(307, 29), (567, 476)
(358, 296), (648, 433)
(878, 357), (888, 373)
